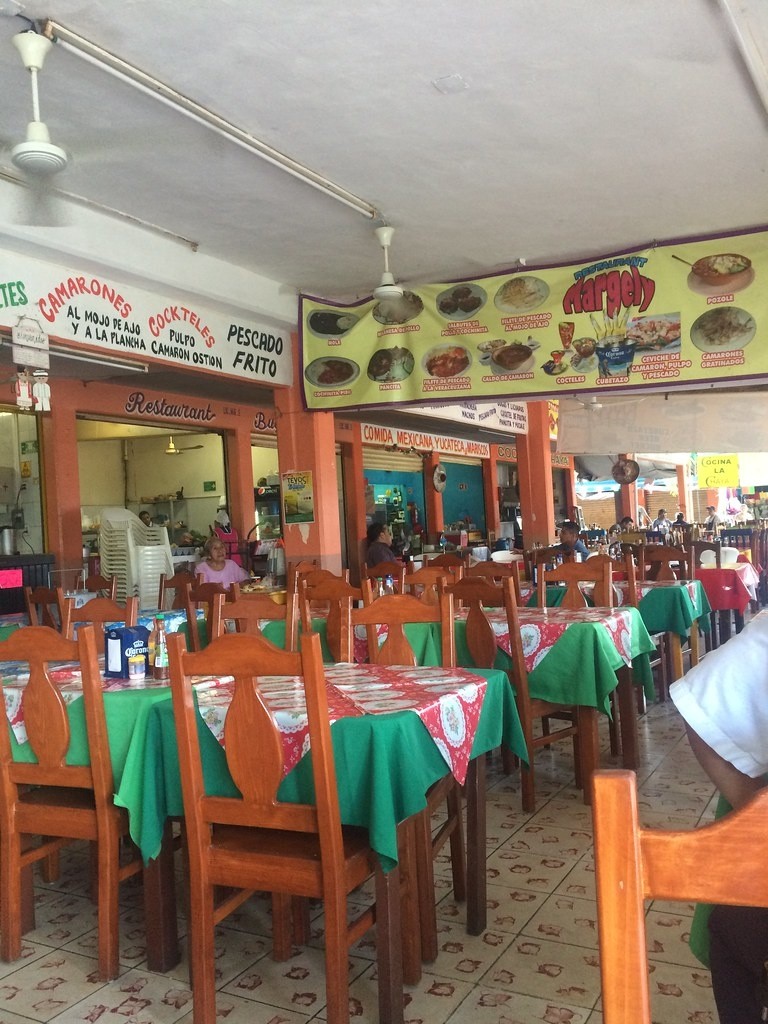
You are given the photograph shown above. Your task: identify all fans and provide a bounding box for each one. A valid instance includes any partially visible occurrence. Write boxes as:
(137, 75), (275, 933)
(372, 220), (405, 303)
(560, 391), (644, 417)
(0, 32), (102, 228)
(152, 434), (204, 455)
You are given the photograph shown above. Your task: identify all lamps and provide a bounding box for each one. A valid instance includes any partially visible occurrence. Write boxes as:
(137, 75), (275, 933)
(43, 20), (379, 223)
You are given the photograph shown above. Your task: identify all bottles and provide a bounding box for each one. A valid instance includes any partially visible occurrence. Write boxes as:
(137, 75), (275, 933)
(148, 615), (169, 680)
(372, 575), (394, 600)
(588, 523), (736, 546)
(599, 540), (623, 562)
(532, 560), (537, 586)
(552, 553), (566, 587)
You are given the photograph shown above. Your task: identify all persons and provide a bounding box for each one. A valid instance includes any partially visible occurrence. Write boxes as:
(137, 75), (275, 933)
(735, 504), (753, 521)
(704, 505), (721, 530)
(139, 511), (159, 527)
(366, 524), (422, 592)
(194, 538), (252, 634)
(669, 609), (768, 809)
(672, 512), (690, 530)
(609, 516), (634, 533)
(653, 509), (672, 529)
(526, 522), (590, 564)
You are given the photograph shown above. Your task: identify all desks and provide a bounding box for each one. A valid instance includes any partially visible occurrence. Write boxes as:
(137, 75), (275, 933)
(177, 596), (440, 667)
(147, 663), (529, 984)
(415, 606), (658, 804)
(4, 604), (207, 654)
(533, 578), (706, 702)
(0, 660), (232, 973)
(612, 561), (756, 637)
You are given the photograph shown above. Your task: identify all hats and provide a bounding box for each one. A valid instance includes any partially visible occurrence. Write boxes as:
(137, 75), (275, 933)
(706, 506), (716, 511)
(556, 519), (581, 532)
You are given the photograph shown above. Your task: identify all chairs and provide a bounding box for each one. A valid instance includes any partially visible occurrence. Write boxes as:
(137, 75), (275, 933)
(0, 507), (768, 1024)
